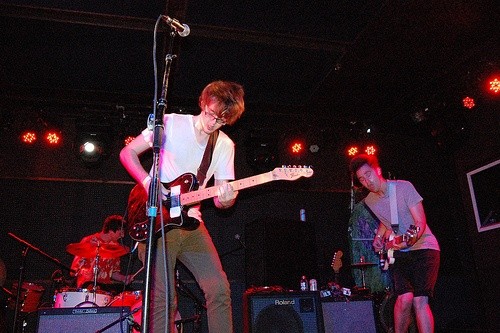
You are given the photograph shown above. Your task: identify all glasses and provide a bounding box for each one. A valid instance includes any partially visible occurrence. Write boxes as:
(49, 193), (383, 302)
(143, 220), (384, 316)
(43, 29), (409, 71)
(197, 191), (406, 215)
(205, 105), (227, 126)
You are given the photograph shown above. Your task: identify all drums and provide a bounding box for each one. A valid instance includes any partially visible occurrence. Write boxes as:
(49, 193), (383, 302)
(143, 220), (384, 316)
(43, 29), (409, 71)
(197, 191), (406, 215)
(53, 288), (113, 308)
(105, 290), (143, 333)
(6, 282), (45, 312)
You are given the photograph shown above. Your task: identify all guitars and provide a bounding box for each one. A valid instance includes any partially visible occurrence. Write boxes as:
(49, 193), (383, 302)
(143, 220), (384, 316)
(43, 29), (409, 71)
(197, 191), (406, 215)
(330, 250), (343, 285)
(380, 223), (420, 270)
(126, 164), (313, 244)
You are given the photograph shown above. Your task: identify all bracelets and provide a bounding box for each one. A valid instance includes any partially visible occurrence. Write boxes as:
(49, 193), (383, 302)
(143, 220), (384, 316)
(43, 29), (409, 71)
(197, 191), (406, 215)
(143, 176), (151, 185)
(377, 235), (382, 240)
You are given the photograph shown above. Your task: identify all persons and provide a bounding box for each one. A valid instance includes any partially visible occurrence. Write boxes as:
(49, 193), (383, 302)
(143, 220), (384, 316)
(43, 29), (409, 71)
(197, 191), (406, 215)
(351, 154), (440, 333)
(119, 80), (244, 333)
(70, 215), (134, 292)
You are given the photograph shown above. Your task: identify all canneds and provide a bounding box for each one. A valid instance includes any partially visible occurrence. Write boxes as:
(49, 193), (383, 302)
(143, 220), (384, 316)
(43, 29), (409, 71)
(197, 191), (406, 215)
(310, 278), (317, 291)
(300, 208), (305, 222)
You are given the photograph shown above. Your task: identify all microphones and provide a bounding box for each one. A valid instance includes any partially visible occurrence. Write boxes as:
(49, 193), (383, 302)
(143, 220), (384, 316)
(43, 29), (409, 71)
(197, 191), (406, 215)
(73, 258), (87, 279)
(161, 16), (190, 37)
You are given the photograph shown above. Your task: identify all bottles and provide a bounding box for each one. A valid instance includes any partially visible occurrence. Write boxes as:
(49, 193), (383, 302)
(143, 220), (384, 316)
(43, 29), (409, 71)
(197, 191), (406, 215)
(300, 275), (308, 291)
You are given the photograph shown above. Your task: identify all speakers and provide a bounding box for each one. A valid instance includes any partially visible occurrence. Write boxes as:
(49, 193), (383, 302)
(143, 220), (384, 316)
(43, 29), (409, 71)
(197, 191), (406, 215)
(245, 214), (381, 333)
(33, 306), (133, 333)
